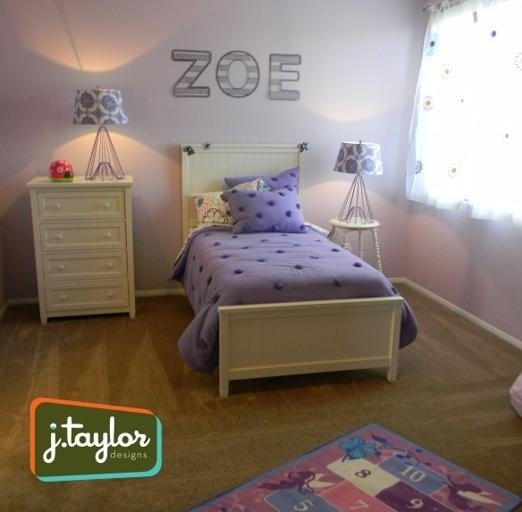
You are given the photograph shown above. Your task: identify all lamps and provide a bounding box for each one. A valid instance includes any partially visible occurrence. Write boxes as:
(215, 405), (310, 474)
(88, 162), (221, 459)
(333, 140), (382, 224)
(72, 86), (129, 181)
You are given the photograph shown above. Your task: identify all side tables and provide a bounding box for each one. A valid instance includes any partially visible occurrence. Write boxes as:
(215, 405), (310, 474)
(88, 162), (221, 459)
(328, 218), (383, 274)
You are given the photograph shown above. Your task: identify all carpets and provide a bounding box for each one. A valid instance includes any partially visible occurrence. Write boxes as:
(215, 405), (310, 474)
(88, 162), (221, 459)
(185, 423), (522, 512)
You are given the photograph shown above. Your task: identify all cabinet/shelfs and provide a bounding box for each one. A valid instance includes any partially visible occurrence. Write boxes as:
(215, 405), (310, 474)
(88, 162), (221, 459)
(27, 176), (135, 325)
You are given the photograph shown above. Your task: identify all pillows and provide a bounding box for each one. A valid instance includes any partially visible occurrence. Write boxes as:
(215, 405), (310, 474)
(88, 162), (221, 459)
(189, 166), (310, 234)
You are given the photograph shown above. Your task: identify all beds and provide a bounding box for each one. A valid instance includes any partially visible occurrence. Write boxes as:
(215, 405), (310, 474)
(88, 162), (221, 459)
(168, 143), (418, 399)
